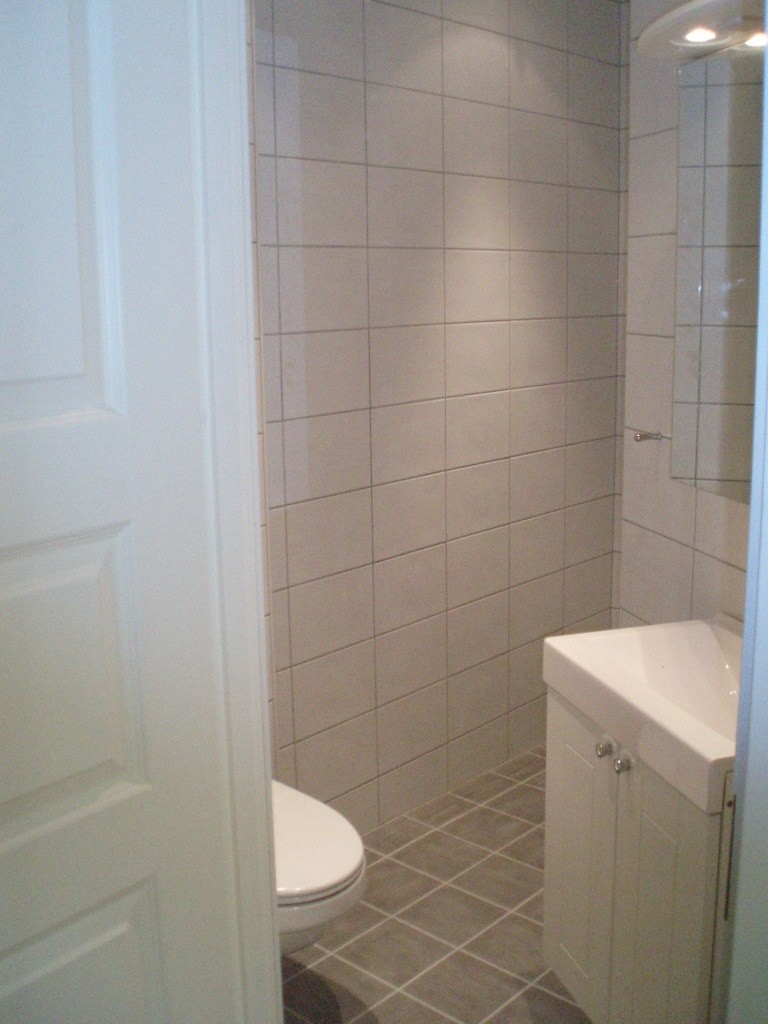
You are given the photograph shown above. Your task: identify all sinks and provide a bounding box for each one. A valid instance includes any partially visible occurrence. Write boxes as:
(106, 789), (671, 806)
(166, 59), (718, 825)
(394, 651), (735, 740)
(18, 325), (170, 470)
(542, 606), (749, 816)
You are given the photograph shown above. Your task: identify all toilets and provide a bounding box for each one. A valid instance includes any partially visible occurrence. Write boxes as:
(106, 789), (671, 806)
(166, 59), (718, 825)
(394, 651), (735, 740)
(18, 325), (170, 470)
(273, 780), (369, 955)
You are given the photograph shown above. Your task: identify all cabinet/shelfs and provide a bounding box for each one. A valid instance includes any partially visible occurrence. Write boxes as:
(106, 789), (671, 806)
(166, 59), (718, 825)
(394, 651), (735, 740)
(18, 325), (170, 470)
(541, 685), (735, 1023)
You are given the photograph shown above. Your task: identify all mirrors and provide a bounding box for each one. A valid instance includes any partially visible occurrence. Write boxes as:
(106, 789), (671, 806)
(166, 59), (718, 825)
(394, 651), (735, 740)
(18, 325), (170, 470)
(668, 29), (768, 505)
